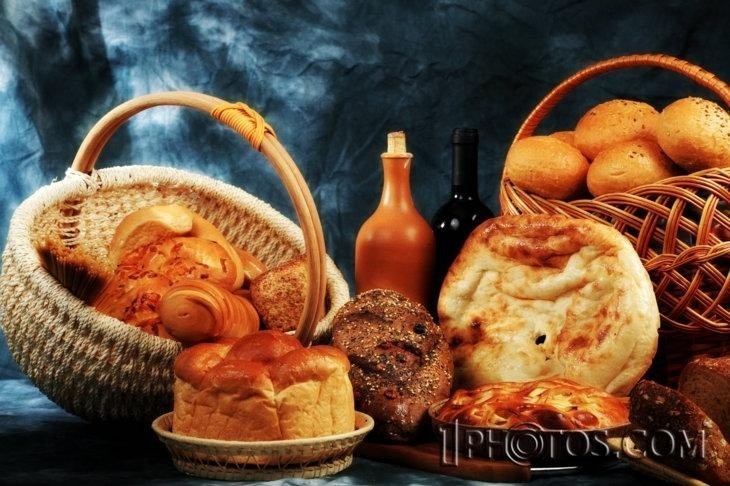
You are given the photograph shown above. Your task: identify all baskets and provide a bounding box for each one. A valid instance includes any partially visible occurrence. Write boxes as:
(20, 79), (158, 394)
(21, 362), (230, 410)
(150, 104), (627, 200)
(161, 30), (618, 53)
(1, 91), (351, 427)
(499, 55), (730, 340)
(152, 412), (375, 481)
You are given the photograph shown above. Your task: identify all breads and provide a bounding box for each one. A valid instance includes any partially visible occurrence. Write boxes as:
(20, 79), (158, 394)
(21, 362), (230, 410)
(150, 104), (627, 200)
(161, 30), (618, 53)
(107, 96), (730, 486)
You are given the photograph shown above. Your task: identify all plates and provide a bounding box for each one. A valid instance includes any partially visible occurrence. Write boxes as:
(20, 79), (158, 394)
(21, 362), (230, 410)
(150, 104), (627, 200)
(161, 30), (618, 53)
(427, 395), (634, 461)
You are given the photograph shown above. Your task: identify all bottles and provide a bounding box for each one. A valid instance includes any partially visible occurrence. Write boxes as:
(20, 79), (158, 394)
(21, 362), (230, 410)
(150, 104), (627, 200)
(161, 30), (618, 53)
(353, 128), (436, 314)
(430, 126), (496, 317)
